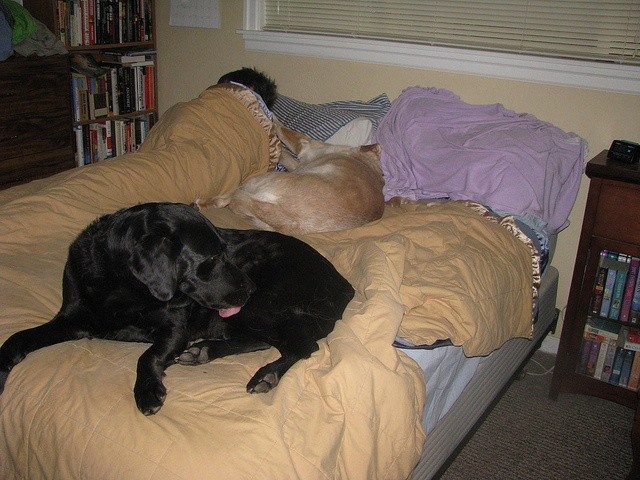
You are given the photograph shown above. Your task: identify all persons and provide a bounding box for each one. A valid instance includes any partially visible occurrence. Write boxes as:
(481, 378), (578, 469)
(212, 64), (278, 112)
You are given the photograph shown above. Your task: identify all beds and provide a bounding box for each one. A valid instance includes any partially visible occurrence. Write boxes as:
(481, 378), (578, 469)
(0, 85), (589, 480)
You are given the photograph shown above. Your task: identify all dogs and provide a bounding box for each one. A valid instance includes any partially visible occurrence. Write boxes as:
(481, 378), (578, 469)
(194, 125), (385, 235)
(0, 202), (354, 416)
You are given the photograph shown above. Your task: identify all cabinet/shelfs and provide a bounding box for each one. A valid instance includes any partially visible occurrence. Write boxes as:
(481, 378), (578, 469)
(54, 0), (160, 168)
(0, 54), (76, 191)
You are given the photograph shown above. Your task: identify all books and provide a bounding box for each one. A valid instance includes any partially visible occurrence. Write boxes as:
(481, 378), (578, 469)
(573, 250), (639, 392)
(58, 1), (157, 166)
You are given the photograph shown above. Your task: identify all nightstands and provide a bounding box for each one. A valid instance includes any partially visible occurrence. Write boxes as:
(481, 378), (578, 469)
(549, 149), (640, 399)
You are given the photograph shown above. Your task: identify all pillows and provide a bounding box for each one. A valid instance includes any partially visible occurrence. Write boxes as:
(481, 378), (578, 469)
(375, 85), (589, 231)
(270, 92), (373, 175)
